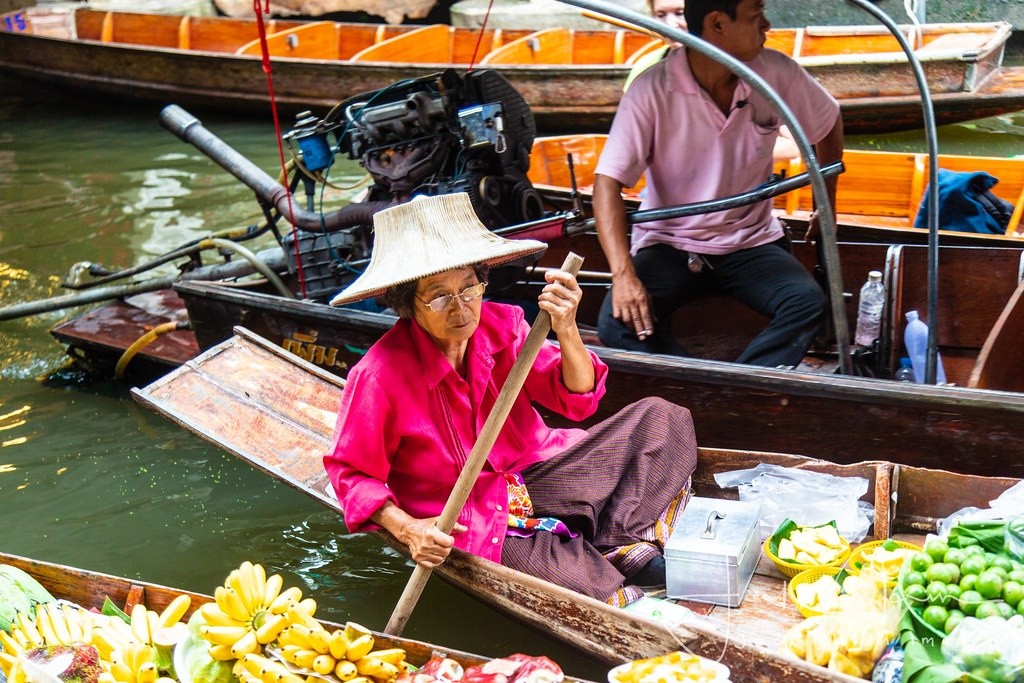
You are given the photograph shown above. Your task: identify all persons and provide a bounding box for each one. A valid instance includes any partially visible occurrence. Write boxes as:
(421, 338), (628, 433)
(596, 0), (843, 367)
(621, 0), (808, 194)
(320, 192), (697, 610)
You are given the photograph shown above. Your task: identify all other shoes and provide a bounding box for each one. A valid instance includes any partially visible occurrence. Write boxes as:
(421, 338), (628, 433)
(621, 556), (668, 588)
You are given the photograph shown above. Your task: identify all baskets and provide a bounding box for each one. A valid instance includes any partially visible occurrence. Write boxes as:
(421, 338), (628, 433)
(788, 567), (880, 619)
(764, 524), (850, 577)
(849, 540), (924, 587)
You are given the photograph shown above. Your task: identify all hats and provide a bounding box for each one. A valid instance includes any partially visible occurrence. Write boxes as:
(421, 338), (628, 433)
(328, 192), (548, 306)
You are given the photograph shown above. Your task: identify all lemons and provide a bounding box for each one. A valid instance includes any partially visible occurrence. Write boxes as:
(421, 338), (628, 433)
(904, 537), (1024, 683)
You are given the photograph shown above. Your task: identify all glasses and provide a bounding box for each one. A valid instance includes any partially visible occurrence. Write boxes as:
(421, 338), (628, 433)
(413, 273), (488, 312)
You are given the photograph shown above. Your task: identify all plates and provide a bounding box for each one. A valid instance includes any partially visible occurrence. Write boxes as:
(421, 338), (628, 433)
(607, 655), (730, 683)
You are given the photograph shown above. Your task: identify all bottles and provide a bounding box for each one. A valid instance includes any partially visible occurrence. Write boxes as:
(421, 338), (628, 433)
(904, 310), (947, 385)
(855, 271), (885, 346)
(895, 357), (916, 384)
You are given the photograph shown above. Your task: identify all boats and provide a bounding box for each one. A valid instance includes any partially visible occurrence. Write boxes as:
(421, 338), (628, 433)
(0, 0), (1024, 132)
(47, 66), (1024, 478)
(527, 132), (1024, 247)
(130, 326), (1024, 683)
(0, 551), (604, 683)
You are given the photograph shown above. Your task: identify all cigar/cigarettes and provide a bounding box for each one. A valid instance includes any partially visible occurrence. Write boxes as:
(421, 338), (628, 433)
(637, 331), (646, 336)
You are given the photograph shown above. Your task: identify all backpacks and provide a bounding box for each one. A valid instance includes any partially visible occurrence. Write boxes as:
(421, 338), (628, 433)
(916, 169), (1014, 235)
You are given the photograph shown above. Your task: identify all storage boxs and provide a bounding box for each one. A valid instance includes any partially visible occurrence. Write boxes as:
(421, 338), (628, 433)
(662, 495), (763, 609)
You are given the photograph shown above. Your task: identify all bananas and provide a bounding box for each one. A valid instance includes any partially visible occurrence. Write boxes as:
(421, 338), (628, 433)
(0, 560), (409, 683)
